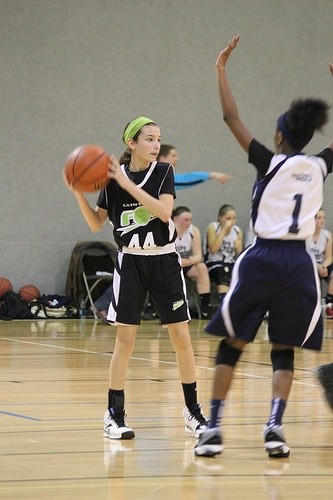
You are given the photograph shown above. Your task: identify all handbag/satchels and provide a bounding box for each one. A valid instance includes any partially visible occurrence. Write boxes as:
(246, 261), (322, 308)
(0, 291), (73, 320)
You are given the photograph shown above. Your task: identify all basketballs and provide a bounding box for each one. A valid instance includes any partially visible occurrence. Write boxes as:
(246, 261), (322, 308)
(18, 285), (41, 302)
(63, 142), (114, 193)
(0, 277), (13, 297)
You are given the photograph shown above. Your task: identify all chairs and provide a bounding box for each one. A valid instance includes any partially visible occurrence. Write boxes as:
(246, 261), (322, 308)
(68, 241), (203, 320)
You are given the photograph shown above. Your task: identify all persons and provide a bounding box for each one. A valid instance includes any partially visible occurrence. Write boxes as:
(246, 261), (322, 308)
(63, 116), (209, 440)
(193, 35), (333, 460)
(93, 143), (333, 327)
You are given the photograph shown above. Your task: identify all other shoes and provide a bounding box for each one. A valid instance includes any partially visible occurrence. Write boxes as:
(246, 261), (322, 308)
(89, 302), (111, 324)
(201, 311), (213, 320)
(103, 407), (135, 439)
(193, 428), (223, 456)
(143, 303), (156, 319)
(264, 422), (290, 457)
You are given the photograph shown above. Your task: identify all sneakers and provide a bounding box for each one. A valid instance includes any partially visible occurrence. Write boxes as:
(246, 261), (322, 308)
(183, 403), (208, 433)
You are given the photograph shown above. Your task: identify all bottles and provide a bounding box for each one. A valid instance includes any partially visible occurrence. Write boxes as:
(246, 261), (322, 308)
(80, 299), (87, 319)
